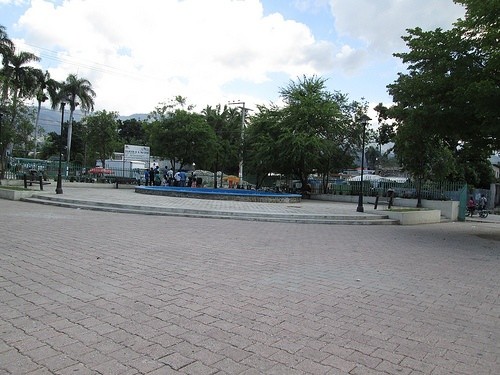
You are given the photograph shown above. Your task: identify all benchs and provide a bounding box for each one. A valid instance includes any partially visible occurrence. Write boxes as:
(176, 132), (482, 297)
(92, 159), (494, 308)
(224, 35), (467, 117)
(368, 195), (393, 210)
(22, 172), (52, 190)
(115, 176), (142, 189)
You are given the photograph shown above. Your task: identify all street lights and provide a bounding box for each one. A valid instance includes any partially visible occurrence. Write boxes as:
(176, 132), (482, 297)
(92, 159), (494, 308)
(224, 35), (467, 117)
(56, 101), (66, 195)
(356, 114), (373, 213)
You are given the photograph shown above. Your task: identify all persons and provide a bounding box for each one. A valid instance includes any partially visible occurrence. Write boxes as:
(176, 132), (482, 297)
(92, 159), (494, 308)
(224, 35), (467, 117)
(236, 185), (251, 190)
(387, 187), (394, 209)
(478, 194), (487, 210)
(273, 183), (311, 200)
(134, 165), (202, 188)
(467, 197), (474, 217)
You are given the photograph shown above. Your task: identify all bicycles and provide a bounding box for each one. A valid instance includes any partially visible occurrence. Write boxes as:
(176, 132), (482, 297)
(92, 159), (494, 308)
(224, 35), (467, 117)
(465, 205), (489, 219)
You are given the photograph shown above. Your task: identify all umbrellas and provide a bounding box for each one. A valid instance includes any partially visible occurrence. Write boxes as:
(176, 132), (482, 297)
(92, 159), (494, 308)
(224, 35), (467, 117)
(91, 167), (115, 174)
